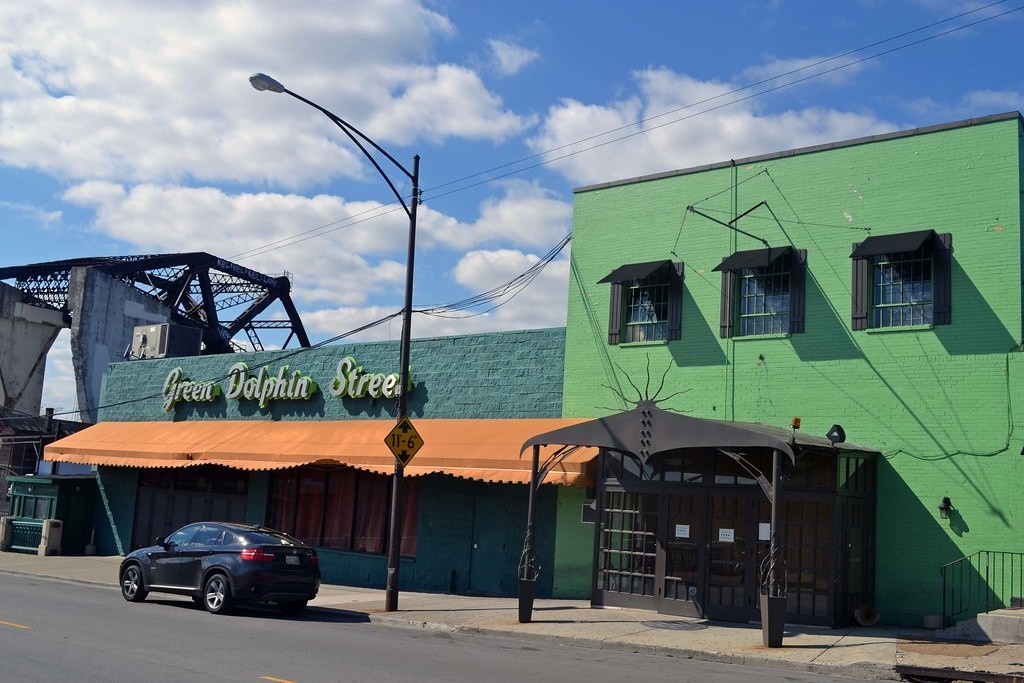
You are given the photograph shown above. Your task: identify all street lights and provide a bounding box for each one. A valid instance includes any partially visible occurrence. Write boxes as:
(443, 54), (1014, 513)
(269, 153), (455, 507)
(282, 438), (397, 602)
(249, 72), (421, 611)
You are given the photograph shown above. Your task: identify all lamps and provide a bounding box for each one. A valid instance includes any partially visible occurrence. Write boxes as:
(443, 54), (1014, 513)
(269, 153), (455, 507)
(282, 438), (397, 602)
(788, 417), (802, 447)
(825, 424), (846, 446)
(937, 497), (954, 520)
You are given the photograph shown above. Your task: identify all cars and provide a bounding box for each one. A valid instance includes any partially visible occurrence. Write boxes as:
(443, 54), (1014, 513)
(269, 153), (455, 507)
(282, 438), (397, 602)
(118, 521), (321, 620)
